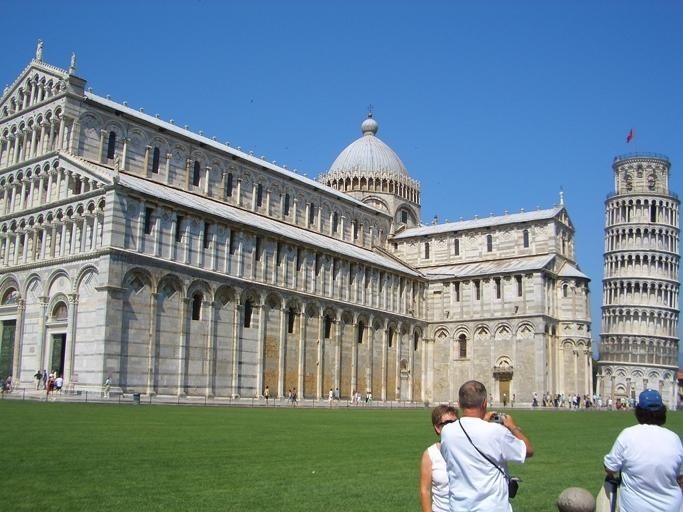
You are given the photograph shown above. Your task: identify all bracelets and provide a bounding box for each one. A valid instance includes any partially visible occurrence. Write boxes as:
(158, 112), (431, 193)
(511, 426), (522, 432)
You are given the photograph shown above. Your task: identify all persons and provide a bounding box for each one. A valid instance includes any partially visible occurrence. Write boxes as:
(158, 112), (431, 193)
(334, 388), (340, 400)
(264, 385), (270, 399)
(328, 388), (334, 400)
(531, 391), (637, 410)
(503, 393), (507, 407)
(0, 373), (13, 397)
(603, 388), (683, 512)
(511, 394), (515, 407)
(419, 405), (460, 512)
(489, 393), (493, 407)
(103, 377), (112, 397)
(353, 390), (373, 404)
(33, 368), (63, 397)
(440, 379), (534, 512)
(287, 388), (298, 405)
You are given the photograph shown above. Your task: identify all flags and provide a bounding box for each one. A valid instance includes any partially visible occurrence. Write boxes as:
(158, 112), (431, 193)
(626, 127), (634, 144)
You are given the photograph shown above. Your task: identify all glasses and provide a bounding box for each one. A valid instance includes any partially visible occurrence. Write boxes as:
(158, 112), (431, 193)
(437, 420), (454, 427)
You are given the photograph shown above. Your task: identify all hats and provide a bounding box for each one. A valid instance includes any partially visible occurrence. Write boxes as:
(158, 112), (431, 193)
(639, 389), (662, 410)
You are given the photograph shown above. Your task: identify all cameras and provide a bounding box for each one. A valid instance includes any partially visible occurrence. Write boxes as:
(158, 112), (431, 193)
(492, 413), (504, 423)
